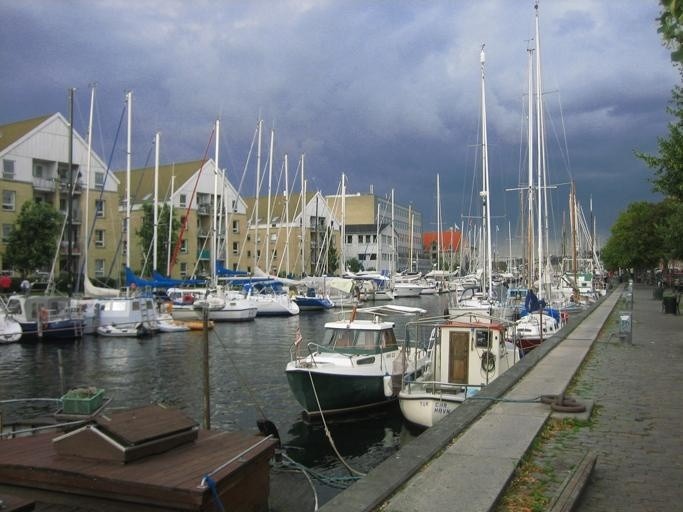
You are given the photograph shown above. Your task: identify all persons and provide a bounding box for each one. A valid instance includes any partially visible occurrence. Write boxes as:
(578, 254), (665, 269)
(20, 278), (31, 294)
(0, 274), (12, 293)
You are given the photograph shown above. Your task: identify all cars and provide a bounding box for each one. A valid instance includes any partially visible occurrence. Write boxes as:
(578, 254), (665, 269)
(635, 266), (683, 291)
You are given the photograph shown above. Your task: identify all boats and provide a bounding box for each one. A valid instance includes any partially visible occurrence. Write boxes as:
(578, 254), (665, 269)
(277, 281), (430, 425)
(0, 394), (107, 441)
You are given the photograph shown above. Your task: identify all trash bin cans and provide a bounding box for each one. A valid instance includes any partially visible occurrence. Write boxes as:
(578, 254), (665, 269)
(662, 295), (681, 314)
